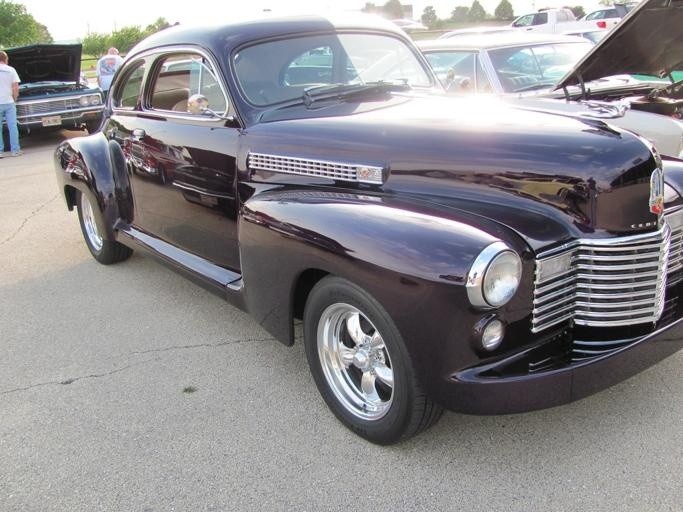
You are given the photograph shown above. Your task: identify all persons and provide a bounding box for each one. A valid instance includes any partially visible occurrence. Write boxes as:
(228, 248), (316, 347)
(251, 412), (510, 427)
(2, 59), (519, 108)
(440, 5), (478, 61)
(95, 46), (125, 102)
(0, 50), (25, 157)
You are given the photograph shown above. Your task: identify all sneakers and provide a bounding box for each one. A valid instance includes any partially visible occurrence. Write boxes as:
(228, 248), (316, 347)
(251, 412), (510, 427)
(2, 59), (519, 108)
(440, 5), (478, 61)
(11, 150), (23, 157)
(0, 153), (5, 158)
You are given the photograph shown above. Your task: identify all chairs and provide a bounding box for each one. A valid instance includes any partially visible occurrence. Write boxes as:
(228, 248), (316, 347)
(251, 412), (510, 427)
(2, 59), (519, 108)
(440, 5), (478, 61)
(149, 82), (225, 114)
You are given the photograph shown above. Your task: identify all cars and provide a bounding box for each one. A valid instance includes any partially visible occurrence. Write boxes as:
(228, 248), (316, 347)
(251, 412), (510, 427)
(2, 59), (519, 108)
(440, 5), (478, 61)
(45, 8), (683, 453)
(488, 168), (592, 225)
(106, 127), (238, 222)
(0, 43), (106, 142)
(383, 1), (683, 164)
